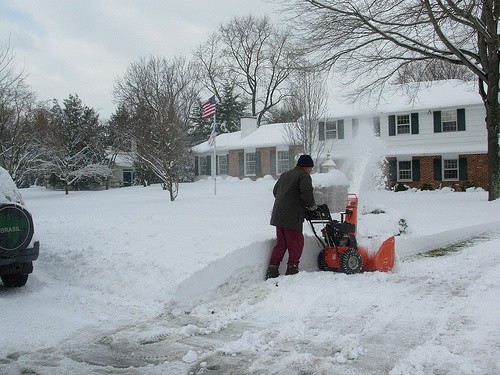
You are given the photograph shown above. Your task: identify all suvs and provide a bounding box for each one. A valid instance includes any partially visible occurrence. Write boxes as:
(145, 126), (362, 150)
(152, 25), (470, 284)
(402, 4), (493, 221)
(0, 164), (40, 289)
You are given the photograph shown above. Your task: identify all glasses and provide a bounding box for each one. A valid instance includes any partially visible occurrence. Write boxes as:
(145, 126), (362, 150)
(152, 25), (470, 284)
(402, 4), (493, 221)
(296, 155), (314, 167)
(308, 168), (313, 171)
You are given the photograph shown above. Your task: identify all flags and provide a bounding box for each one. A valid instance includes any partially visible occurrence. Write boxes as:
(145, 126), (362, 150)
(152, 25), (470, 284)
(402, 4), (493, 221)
(200, 95), (215, 120)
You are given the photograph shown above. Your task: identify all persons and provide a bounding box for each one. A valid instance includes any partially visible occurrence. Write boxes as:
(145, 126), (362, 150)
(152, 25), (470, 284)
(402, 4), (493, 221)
(264, 155), (318, 280)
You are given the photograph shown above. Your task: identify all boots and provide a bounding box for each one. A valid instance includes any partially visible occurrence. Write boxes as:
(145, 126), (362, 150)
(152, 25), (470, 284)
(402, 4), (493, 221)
(265, 265), (280, 280)
(285, 263), (299, 275)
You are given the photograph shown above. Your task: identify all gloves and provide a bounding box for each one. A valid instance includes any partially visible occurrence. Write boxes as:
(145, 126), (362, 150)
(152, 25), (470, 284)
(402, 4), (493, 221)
(307, 204), (318, 210)
